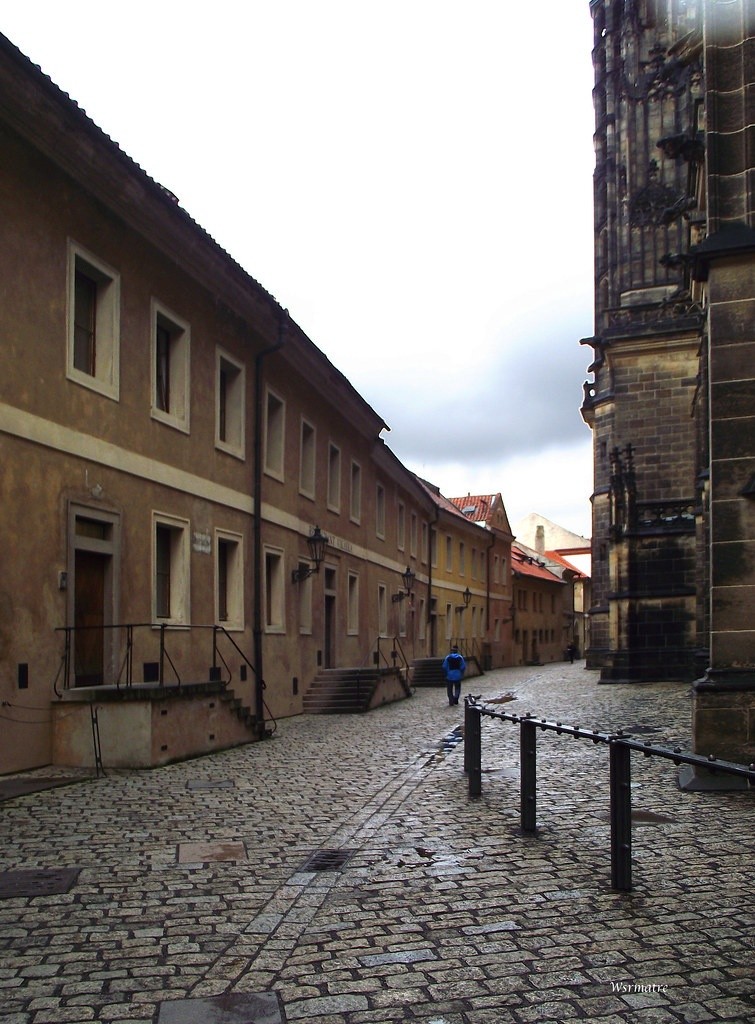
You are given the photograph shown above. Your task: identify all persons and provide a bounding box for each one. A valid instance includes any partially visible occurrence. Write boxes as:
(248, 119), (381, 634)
(567, 642), (576, 664)
(442, 646), (465, 706)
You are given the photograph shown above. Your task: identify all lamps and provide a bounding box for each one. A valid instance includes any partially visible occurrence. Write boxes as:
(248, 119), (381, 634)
(292, 525), (329, 584)
(392, 566), (416, 603)
(502, 604), (517, 624)
(456, 586), (473, 613)
(563, 614), (574, 630)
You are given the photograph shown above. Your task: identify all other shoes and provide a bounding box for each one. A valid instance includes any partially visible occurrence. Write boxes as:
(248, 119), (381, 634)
(453, 698), (458, 705)
(449, 700), (454, 706)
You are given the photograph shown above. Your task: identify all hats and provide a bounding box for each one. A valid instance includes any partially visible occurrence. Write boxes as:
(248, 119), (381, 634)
(450, 646), (458, 650)
